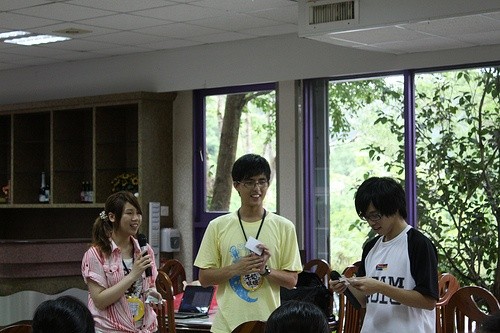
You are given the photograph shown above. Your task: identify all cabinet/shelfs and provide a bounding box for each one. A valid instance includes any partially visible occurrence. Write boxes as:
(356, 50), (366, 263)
(0, 91), (177, 263)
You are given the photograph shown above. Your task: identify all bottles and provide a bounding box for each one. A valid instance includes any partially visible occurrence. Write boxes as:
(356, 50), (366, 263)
(80, 181), (93, 203)
(39, 172), (49, 203)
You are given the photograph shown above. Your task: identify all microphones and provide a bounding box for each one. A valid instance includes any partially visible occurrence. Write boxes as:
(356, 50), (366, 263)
(138, 233), (152, 277)
(330, 270), (360, 310)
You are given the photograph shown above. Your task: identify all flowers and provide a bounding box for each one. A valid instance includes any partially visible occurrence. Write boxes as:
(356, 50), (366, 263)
(111, 172), (139, 192)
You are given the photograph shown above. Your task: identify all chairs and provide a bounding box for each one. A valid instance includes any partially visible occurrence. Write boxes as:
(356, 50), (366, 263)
(153, 260), (187, 333)
(303, 259), (500, 333)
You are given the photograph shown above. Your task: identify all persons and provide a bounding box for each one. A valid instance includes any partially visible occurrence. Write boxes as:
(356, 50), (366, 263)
(81, 189), (168, 333)
(329, 177), (440, 333)
(264, 299), (332, 333)
(193, 154), (304, 332)
(32, 295), (96, 333)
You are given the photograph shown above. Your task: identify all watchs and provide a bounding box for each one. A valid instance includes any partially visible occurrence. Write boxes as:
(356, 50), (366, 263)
(259, 265), (271, 276)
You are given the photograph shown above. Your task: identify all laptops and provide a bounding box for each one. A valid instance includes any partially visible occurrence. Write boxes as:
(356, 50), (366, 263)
(163, 285), (214, 319)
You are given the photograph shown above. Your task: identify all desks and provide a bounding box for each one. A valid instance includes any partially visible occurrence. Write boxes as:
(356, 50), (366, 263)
(165, 313), (338, 333)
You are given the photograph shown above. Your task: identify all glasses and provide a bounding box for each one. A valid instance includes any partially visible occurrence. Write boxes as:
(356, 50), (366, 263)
(359, 212), (384, 221)
(237, 181), (267, 189)
